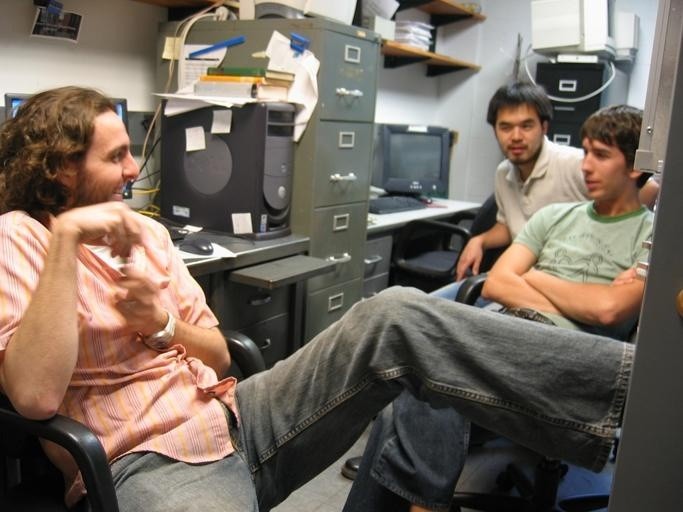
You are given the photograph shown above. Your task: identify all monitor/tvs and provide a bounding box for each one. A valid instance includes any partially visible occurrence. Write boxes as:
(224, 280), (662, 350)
(370, 123), (450, 198)
(4, 92), (132, 200)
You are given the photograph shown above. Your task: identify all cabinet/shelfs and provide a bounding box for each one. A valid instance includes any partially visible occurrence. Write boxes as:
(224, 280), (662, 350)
(153, 0), (482, 369)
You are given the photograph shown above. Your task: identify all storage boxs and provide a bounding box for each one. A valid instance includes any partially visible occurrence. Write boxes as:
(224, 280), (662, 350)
(534, 63), (630, 148)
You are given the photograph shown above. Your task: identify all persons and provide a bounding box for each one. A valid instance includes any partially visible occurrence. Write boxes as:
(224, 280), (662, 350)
(369, 103), (654, 512)
(0, 85), (640, 512)
(338, 83), (664, 481)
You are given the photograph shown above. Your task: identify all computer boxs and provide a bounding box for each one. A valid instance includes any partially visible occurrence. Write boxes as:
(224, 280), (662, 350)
(158, 97), (295, 240)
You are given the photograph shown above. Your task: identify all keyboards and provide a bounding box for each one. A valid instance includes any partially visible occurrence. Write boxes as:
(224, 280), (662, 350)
(369, 194), (424, 215)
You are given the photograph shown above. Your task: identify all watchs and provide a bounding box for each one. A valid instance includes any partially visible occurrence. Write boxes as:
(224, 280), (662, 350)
(143, 313), (176, 351)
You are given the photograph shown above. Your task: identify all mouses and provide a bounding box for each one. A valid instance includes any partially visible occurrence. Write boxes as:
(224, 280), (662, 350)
(179, 236), (213, 255)
(419, 197), (432, 204)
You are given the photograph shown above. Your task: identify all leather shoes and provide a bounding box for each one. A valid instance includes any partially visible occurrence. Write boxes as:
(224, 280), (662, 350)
(341, 456), (363, 480)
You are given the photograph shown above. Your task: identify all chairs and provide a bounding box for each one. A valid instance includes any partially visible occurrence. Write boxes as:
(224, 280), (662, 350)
(0, 327), (268, 512)
(391, 192), (511, 308)
